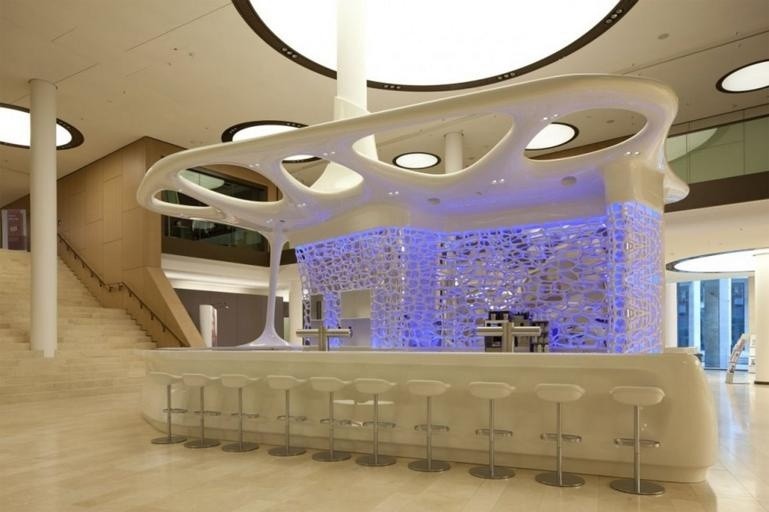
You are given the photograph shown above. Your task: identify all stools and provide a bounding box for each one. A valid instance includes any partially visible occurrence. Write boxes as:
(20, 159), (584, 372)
(308, 375), (349, 462)
(609, 385), (665, 496)
(220, 375), (260, 451)
(266, 375), (306, 456)
(469, 381), (516, 478)
(181, 373), (220, 449)
(354, 378), (396, 467)
(406, 379), (451, 471)
(535, 383), (585, 486)
(150, 370), (186, 445)
